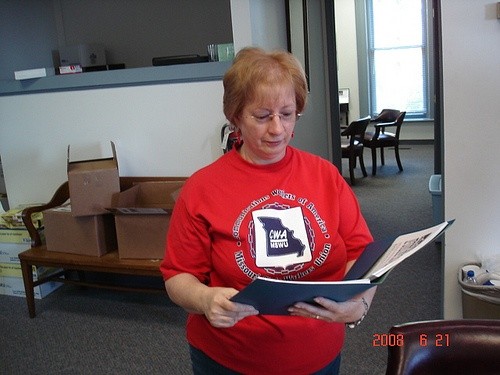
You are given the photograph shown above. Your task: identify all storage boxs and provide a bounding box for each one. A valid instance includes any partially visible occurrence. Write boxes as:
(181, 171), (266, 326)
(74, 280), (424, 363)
(42, 206), (115, 258)
(67, 141), (121, 217)
(104, 181), (186, 260)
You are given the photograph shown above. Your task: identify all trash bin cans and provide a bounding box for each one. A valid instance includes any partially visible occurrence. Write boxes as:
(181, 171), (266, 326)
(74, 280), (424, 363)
(457, 260), (500, 322)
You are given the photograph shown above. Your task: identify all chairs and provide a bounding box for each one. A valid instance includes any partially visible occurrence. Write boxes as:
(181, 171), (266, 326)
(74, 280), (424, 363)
(339, 115), (374, 186)
(365, 108), (406, 177)
(385, 319), (500, 375)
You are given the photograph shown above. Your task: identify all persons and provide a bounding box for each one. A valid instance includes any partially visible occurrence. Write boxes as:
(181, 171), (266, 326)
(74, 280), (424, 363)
(159, 46), (377, 375)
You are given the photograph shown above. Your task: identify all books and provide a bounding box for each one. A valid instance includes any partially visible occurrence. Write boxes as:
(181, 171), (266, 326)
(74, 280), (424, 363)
(229, 218), (456, 315)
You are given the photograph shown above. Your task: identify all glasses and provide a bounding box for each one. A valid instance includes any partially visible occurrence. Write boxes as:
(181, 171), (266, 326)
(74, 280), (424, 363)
(239, 110), (303, 121)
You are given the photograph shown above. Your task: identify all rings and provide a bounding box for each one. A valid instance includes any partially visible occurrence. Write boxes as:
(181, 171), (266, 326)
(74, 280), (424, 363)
(315, 313), (320, 320)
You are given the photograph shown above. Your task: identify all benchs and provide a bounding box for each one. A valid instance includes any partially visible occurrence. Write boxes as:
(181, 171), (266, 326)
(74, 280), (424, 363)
(18, 176), (193, 319)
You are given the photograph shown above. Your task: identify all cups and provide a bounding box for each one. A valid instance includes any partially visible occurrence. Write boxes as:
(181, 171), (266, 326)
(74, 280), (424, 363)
(208, 45), (218, 62)
(218, 43), (233, 62)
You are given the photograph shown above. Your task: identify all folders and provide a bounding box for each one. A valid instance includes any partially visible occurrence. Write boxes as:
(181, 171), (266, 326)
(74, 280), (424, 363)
(229, 219), (455, 315)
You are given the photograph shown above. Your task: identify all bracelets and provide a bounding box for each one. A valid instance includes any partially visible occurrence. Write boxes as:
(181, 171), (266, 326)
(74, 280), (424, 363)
(345, 297), (368, 328)
